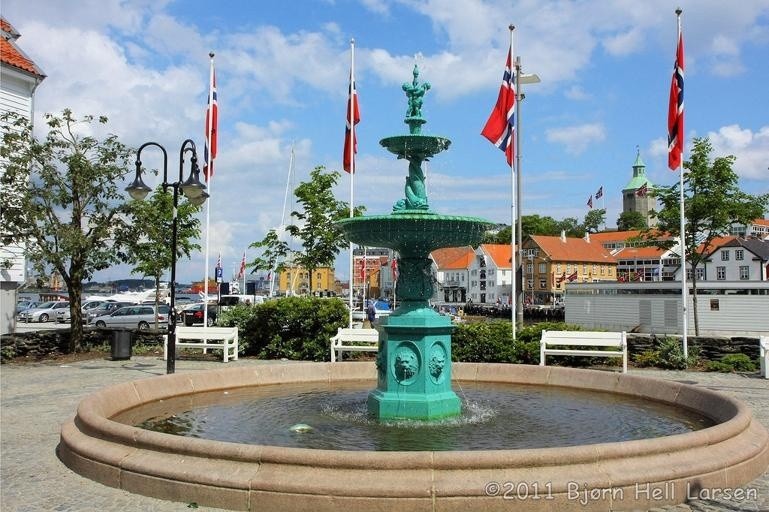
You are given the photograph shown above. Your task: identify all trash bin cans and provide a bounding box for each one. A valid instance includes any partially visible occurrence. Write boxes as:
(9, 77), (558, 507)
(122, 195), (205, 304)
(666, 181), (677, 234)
(111, 330), (131, 360)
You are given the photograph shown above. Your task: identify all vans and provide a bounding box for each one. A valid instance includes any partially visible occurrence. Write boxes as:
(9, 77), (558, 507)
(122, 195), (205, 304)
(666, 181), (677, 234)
(555, 295), (566, 306)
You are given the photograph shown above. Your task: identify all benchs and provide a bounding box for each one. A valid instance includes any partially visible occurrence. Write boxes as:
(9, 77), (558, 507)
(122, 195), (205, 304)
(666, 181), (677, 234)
(539, 329), (627, 372)
(163, 326), (238, 362)
(329, 327), (379, 362)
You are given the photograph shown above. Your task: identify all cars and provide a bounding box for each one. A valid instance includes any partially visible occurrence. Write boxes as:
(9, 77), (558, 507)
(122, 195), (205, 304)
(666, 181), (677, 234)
(16, 293), (267, 335)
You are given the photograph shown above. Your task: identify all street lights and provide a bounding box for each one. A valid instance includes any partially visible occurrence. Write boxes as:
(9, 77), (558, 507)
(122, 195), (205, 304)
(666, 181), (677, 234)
(124, 138), (210, 375)
(366, 274), (369, 309)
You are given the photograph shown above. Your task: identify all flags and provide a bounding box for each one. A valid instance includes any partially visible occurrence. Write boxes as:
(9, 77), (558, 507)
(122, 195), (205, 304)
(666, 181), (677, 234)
(391, 254), (398, 280)
(342, 69), (360, 174)
(216, 252), (221, 267)
(665, 29), (684, 171)
(266, 259), (272, 281)
(567, 272), (577, 282)
(595, 186), (602, 200)
(586, 195), (592, 208)
(479, 44), (516, 172)
(237, 251), (246, 281)
(360, 254), (365, 277)
(636, 182), (647, 197)
(202, 67), (218, 184)
(561, 271), (566, 280)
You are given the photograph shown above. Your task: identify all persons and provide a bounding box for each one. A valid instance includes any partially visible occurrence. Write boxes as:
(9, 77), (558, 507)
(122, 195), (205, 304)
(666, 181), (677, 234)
(366, 302), (378, 329)
(412, 81), (432, 117)
(402, 81), (421, 117)
